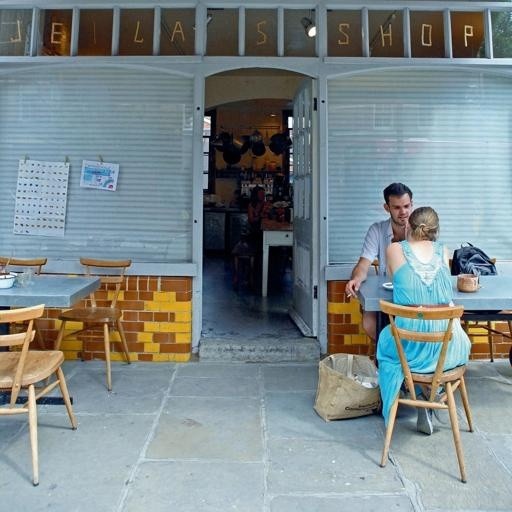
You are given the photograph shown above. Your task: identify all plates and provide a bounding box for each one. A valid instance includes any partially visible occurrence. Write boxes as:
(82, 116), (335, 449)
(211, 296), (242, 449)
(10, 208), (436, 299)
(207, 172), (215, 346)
(383, 281), (393, 290)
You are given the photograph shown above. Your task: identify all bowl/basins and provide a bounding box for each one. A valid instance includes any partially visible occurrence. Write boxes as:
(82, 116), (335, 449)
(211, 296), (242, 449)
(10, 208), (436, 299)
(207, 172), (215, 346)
(0, 275), (16, 287)
(458, 273), (479, 293)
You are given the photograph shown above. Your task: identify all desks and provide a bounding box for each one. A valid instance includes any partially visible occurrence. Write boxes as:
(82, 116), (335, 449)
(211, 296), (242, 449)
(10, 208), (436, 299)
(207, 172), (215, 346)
(262, 222), (294, 297)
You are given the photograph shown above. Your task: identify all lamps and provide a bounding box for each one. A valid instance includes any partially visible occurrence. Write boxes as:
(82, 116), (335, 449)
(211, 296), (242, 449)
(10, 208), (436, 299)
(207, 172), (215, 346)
(192, 8), (213, 31)
(300, 9), (318, 38)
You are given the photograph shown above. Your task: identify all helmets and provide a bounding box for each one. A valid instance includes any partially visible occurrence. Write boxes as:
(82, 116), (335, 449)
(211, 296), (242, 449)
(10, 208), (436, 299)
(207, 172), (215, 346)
(269, 134), (292, 155)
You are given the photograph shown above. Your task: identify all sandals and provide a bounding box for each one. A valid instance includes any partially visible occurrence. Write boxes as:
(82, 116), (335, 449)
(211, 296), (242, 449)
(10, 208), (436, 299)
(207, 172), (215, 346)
(416, 393), (451, 435)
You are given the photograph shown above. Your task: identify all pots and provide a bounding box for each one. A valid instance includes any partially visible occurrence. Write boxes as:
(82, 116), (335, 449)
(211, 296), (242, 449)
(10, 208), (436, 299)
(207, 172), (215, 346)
(214, 126), (287, 161)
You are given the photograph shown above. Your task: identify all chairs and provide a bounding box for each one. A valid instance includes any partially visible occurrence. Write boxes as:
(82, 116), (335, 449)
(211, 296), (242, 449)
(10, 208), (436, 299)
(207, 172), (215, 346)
(379, 299), (475, 483)
(0, 305), (78, 486)
(43, 257), (132, 391)
(360, 257), (380, 356)
(0, 256), (47, 350)
(448, 259), (497, 362)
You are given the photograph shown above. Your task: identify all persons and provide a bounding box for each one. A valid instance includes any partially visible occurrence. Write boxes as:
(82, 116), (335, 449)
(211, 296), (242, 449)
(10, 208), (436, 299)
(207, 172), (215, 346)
(345, 183), (471, 435)
(248, 186), (265, 227)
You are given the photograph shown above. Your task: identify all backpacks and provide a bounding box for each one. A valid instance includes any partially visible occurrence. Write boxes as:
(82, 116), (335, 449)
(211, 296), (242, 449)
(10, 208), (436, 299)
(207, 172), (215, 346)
(451, 241), (503, 315)
